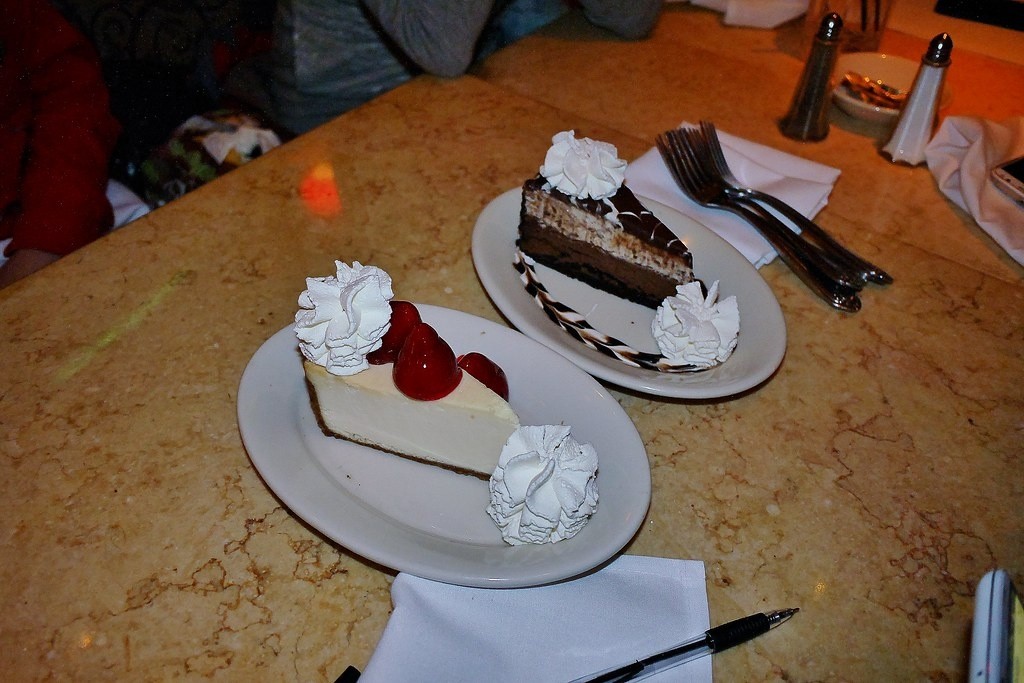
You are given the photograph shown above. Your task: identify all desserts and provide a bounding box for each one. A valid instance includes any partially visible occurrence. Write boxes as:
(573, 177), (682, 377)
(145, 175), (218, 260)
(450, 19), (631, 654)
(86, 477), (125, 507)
(293, 130), (742, 541)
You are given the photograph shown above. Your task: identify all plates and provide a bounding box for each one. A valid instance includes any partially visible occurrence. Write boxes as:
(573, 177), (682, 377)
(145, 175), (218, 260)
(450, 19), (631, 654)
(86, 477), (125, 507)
(235, 300), (655, 592)
(471, 180), (789, 402)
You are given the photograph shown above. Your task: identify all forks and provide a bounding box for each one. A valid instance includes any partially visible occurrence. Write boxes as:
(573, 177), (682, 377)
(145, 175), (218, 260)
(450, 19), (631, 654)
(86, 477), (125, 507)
(654, 118), (894, 317)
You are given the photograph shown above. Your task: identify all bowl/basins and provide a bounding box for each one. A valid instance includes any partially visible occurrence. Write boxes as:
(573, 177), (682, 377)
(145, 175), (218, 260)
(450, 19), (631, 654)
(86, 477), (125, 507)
(830, 51), (953, 129)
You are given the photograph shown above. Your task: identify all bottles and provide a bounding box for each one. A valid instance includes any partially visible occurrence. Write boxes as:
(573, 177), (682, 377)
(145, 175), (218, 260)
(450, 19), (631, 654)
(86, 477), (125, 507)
(780, 12), (843, 144)
(882, 29), (955, 167)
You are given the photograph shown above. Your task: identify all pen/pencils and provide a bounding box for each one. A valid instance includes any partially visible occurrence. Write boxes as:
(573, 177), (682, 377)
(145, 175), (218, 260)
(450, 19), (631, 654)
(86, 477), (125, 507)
(568, 608), (808, 683)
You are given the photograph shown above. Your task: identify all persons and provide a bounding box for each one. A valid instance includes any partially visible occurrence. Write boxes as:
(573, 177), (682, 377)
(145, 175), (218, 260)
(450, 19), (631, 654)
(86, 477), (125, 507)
(0, 0), (120, 294)
(282, 0), (670, 140)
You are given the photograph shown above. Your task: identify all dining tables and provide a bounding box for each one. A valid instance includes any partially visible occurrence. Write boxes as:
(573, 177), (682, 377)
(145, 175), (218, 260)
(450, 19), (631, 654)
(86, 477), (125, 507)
(3, 1), (1024, 683)
